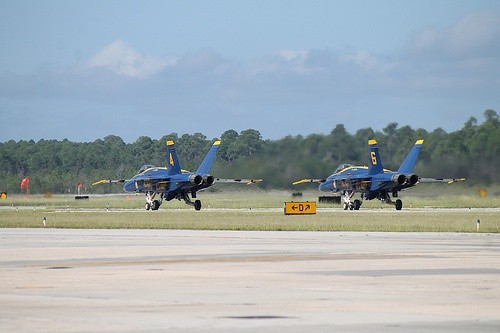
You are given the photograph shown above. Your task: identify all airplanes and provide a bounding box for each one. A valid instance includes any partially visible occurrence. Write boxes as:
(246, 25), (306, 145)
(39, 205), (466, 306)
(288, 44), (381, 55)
(91, 140), (264, 211)
(291, 139), (467, 211)
